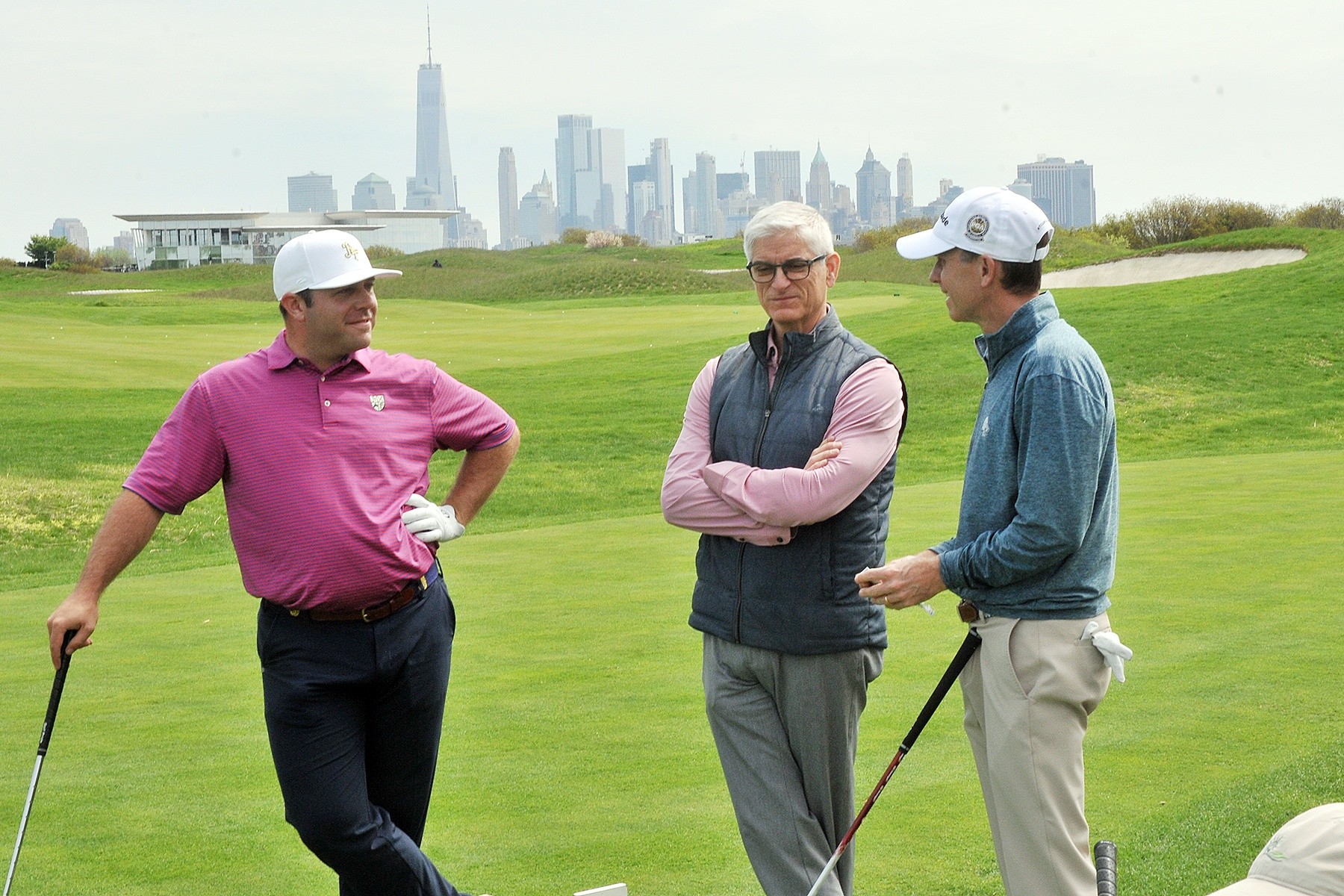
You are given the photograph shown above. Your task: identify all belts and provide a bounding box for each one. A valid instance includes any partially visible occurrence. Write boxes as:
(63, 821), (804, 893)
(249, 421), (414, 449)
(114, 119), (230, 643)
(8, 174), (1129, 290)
(262, 561), (438, 624)
(956, 600), (978, 623)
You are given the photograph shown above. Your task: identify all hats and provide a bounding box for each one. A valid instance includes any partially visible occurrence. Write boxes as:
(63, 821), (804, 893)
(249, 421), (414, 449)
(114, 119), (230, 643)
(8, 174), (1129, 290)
(895, 186), (1054, 263)
(1206, 803), (1344, 896)
(272, 230), (402, 302)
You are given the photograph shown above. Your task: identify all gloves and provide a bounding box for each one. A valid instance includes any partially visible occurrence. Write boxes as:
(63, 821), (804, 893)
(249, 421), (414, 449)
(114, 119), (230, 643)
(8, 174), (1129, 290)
(1080, 620), (1133, 683)
(401, 494), (464, 543)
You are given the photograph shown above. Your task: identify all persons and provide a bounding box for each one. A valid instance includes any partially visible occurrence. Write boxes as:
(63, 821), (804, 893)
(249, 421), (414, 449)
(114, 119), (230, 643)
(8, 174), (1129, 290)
(660, 202), (906, 895)
(46, 231), (520, 896)
(854, 189), (1119, 896)
(432, 260), (441, 267)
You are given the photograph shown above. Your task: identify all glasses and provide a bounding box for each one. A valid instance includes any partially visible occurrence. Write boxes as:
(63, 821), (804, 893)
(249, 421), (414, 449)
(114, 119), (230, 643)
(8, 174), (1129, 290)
(746, 253), (828, 283)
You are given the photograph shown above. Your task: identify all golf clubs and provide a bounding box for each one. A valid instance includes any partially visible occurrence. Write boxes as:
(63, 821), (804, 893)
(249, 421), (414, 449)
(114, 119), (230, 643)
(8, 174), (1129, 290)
(2, 627), (77, 896)
(807, 630), (982, 896)
(1094, 839), (1117, 896)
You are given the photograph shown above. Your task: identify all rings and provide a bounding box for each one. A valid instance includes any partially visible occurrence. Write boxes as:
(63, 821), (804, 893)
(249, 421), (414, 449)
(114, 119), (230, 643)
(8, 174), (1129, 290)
(885, 594), (895, 606)
